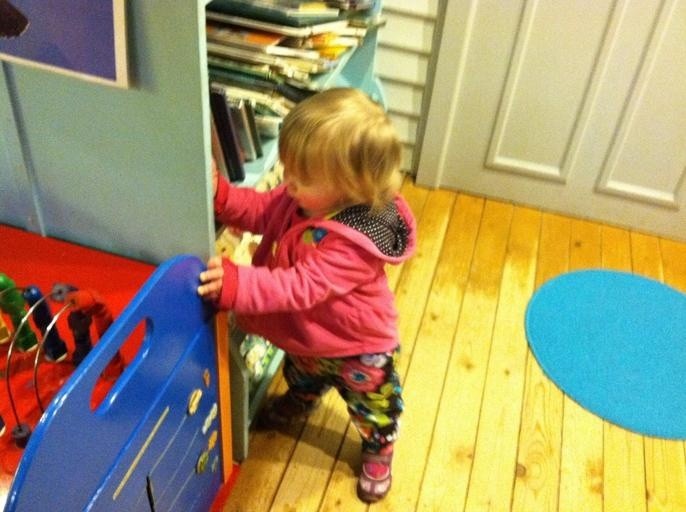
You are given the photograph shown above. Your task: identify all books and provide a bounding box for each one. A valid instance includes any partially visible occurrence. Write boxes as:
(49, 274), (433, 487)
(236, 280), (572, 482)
(205, 0), (384, 180)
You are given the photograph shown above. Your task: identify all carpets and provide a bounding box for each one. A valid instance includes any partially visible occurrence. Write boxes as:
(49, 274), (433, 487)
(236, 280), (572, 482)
(525, 268), (686, 442)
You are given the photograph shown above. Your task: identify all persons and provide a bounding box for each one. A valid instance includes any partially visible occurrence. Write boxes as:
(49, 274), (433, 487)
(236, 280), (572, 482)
(197, 88), (417, 503)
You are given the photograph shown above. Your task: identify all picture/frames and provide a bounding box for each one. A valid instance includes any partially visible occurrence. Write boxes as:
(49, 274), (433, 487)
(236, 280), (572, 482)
(1, 0), (131, 90)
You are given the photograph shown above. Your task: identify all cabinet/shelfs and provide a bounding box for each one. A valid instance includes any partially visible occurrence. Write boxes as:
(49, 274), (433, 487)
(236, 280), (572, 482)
(198, 0), (389, 462)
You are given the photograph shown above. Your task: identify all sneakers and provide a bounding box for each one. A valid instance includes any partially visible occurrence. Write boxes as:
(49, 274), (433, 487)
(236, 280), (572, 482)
(358, 445), (393, 502)
(260, 396), (318, 429)
